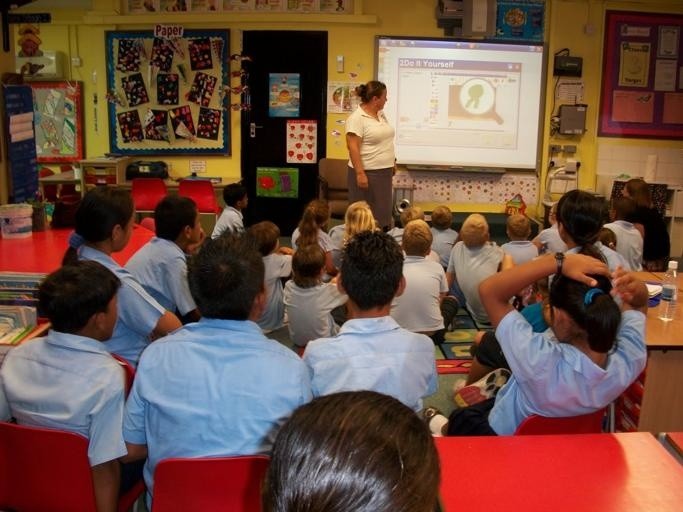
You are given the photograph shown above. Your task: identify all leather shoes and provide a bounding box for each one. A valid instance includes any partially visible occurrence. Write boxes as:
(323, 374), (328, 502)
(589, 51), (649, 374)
(421, 406), (449, 436)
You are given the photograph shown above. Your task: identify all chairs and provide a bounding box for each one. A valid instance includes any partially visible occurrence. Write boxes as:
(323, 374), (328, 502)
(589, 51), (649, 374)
(319, 158), (352, 219)
(150, 455), (274, 511)
(111, 354), (136, 401)
(0, 422), (97, 512)
(130, 177), (171, 214)
(139, 217), (156, 234)
(178, 180), (222, 225)
(39, 168), (72, 205)
(60, 166), (81, 199)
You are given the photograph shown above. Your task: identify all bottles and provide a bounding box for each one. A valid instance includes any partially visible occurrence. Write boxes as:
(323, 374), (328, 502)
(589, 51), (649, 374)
(658, 260), (679, 322)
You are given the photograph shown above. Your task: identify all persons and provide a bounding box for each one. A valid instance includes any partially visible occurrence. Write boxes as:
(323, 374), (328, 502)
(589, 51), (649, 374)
(346, 80), (397, 233)
(2, 180), (669, 512)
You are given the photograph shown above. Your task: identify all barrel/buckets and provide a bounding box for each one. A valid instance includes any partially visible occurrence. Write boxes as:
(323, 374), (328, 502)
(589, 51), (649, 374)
(0, 204), (33, 240)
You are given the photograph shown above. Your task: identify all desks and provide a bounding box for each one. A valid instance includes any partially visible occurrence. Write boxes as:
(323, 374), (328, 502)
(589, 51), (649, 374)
(39, 168), (81, 201)
(0, 222), (156, 282)
(428, 431), (683, 512)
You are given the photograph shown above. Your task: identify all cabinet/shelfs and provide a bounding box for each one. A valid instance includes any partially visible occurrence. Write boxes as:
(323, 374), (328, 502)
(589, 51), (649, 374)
(599, 175), (683, 260)
(614, 271), (682, 432)
(81, 157), (241, 234)
